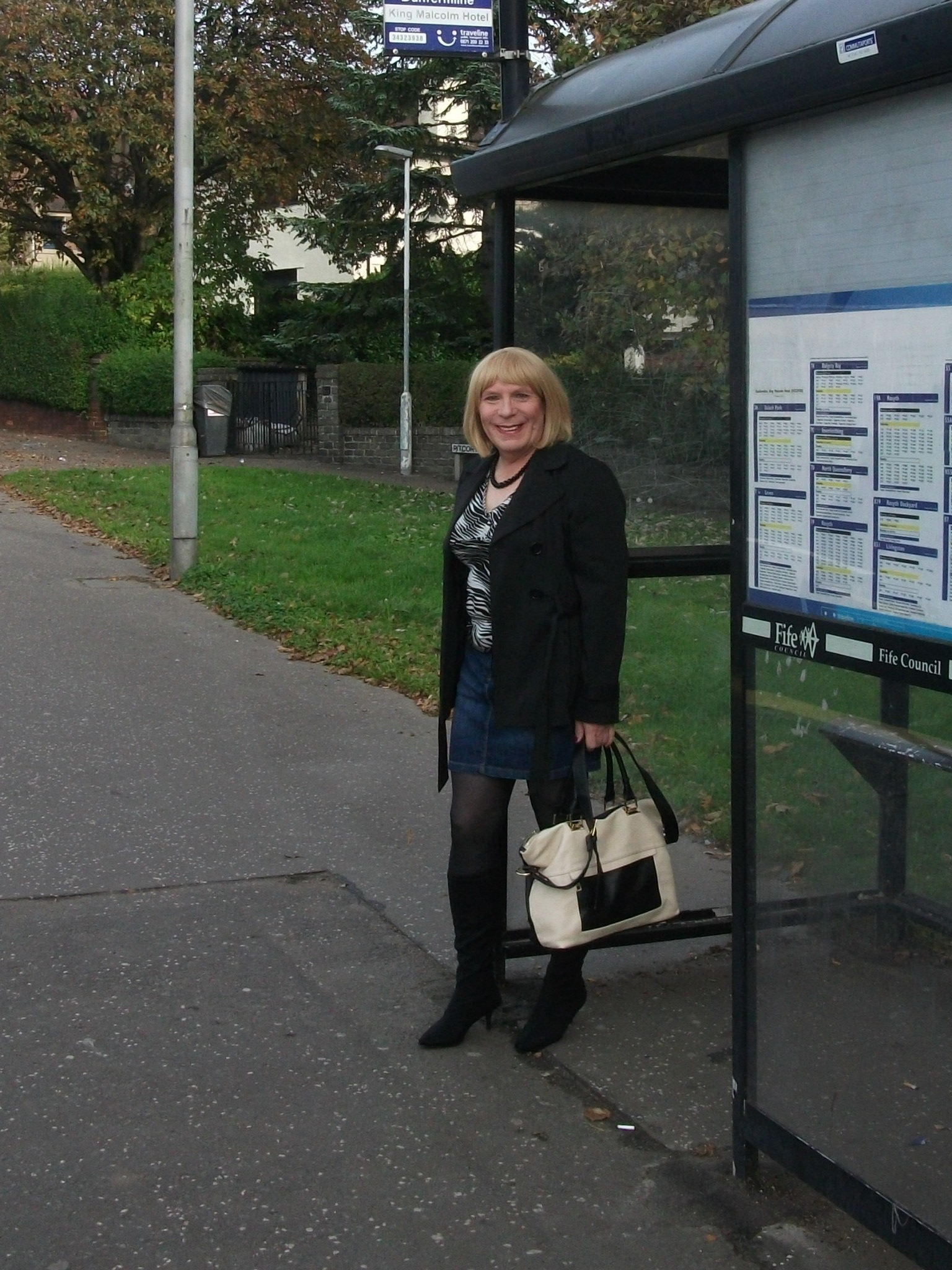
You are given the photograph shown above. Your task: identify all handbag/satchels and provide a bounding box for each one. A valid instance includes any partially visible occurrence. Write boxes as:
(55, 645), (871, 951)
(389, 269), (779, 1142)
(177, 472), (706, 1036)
(520, 797), (681, 954)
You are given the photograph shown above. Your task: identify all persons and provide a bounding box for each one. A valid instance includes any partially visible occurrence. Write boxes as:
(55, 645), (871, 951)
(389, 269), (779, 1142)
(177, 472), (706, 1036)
(419, 346), (627, 1057)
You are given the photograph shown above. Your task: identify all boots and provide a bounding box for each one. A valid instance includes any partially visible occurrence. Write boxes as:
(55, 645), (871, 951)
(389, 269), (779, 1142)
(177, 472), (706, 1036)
(512, 942), (589, 1054)
(419, 864), (511, 1045)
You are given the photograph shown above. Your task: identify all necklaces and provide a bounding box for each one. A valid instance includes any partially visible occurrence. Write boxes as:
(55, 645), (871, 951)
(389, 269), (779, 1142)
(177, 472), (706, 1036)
(489, 450), (535, 489)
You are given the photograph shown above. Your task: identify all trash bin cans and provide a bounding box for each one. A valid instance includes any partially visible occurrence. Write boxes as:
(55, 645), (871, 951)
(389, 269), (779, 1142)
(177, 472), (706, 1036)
(193, 384), (232, 458)
